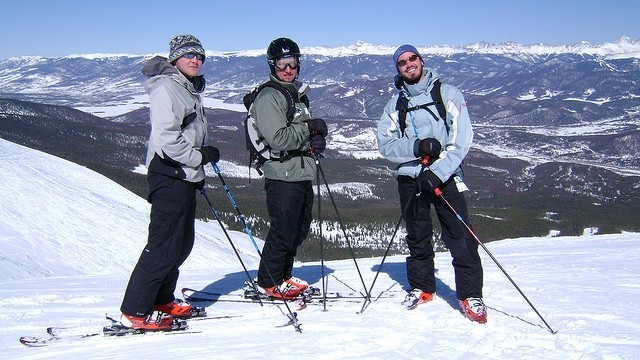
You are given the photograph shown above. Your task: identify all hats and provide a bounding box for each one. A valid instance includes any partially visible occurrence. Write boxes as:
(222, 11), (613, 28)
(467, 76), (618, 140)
(168, 34), (205, 64)
(393, 44), (424, 72)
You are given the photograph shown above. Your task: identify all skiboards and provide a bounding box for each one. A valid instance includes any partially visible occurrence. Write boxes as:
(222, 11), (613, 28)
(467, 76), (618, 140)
(19, 299), (307, 347)
(182, 283), (406, 302)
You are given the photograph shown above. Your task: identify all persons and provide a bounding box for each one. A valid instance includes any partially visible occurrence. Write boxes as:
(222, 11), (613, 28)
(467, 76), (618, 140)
(105, 34), (219, 332)
(243, 38), (328, 303)
(375, 43), (490, 323)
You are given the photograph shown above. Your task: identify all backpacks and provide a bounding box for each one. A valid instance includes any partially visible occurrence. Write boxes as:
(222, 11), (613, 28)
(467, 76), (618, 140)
(243, 82), (295, 184)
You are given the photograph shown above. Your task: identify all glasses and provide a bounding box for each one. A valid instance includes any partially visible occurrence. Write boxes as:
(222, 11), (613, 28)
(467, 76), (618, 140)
(182, 52), (203, 60)
(269, 55), (299, 70)
(396, 54), (419, 67)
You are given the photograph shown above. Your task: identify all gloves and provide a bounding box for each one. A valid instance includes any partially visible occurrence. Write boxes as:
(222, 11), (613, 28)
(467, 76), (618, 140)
(416, 170), (441, 193)
(305, 118), (328, 137)
(198, 145), (219, 165)
(416, 137), (441, 159)
(310, 136), (325, 153)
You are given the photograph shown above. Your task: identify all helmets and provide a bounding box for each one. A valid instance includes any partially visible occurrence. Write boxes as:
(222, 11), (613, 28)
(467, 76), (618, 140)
(267, 38), (301, 76)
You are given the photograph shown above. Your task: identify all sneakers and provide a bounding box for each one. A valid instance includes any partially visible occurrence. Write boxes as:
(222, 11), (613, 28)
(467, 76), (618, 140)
(460, 296), (486, 323)
(121, 311), (173, 329)
(153, 298), (193, 319)
(257, 279), (301, 299)
(399, 288), (436, 310)
(284, 276), (308, 291)
